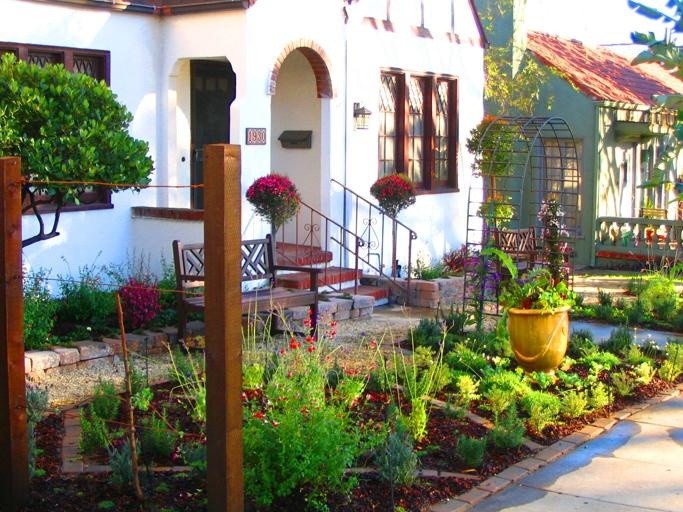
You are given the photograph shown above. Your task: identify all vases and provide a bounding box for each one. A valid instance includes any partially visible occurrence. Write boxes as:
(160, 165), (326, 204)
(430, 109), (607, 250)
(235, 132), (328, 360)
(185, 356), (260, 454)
(507, 305), (571, 371)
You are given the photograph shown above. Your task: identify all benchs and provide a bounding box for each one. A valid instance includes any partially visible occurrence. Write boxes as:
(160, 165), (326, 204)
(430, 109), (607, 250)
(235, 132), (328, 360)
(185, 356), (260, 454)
(171, 233), (322, 351)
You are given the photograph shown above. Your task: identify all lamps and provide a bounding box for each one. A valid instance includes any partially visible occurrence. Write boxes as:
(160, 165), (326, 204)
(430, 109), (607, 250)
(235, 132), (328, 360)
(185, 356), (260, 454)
(353, 102), (371, 130)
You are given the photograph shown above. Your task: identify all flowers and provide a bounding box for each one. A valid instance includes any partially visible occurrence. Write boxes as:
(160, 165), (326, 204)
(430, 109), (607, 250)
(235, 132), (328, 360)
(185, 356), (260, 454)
(368, 172), (417, 218)
(244, 172), (302, 236)
(478, 247), (576, 329)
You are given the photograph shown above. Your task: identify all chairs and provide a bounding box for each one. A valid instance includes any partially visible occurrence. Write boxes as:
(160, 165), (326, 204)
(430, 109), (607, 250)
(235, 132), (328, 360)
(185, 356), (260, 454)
(492, 226), (545, 298)
